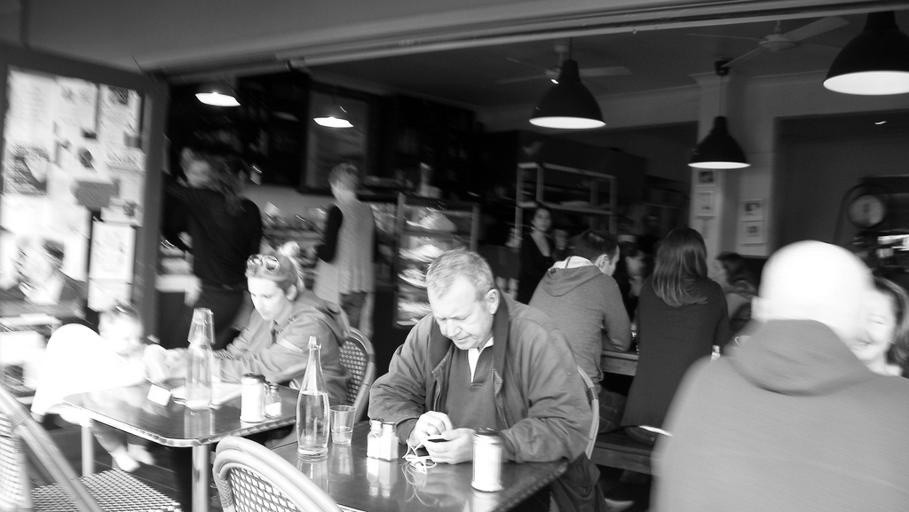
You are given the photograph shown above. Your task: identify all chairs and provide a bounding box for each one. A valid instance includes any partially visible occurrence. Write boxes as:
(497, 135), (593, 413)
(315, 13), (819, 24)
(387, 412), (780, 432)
(30, 323), (120, 475)
(577, 368), (601, 459)
(0, 385), (177, 511)
(212, 435), (345, 512)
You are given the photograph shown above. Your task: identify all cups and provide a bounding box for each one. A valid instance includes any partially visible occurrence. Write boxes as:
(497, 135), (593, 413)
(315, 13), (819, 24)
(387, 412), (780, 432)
(329, 445), (355, 482)
(187, 307), (216, 345)
(329, 404), (358, 445)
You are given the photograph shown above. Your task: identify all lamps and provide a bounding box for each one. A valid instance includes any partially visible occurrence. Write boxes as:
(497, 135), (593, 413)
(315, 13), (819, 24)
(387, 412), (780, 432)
(687, 59), (752, 171)
(529, 37), (608, 130)
(194, 81), (243, 110)
(313, 60), (355, 130)
(821, 11), (909, 97)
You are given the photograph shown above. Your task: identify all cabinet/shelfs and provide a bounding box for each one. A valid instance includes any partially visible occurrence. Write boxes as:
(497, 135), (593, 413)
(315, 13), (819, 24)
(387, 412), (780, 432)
(388, 190), (481, 368)
(507, 159), (620, 304)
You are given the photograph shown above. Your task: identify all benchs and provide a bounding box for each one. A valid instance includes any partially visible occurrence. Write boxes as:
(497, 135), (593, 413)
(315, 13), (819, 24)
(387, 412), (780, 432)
(593, 430), (660, 476)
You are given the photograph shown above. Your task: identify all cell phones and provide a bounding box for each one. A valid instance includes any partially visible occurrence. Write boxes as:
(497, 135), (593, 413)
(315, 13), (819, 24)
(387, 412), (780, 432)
(423, 432), (448, 446)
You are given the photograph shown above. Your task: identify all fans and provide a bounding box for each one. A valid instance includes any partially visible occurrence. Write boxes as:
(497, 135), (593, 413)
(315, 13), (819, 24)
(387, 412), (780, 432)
(686, 18), (850, 69)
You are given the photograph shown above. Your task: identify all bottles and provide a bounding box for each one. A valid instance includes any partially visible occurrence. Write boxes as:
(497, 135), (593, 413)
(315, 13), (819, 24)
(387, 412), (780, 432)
(239, 372), (282, 424)
(184, 311), (215, 410)
(297, 455), (328, 493)
(184, 407), (216, 439)
(366, 416), (400, 462)
(366, 458), (399, 499)
(469, 424), (505, 494)
(295, 335), (331, 459)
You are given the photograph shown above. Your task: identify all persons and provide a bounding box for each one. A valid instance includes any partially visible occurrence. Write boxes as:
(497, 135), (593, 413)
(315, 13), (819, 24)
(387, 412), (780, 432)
(525, 228), (632, 444)
(366, 245), (608, 512)
(516, 198), (759, 352)
(306, 162), (379, 328)
(621, 230), (739, 445)
(853, 268), (909, 378)
(87, 302), (155, 473)
(212, 247), (352, 451)
(647, 238), (908, 512)
(158, 152), (263, 348)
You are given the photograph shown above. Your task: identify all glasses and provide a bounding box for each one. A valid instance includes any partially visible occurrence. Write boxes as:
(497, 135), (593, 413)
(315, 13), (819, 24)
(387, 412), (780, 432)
(245, 253), (280, 274)
